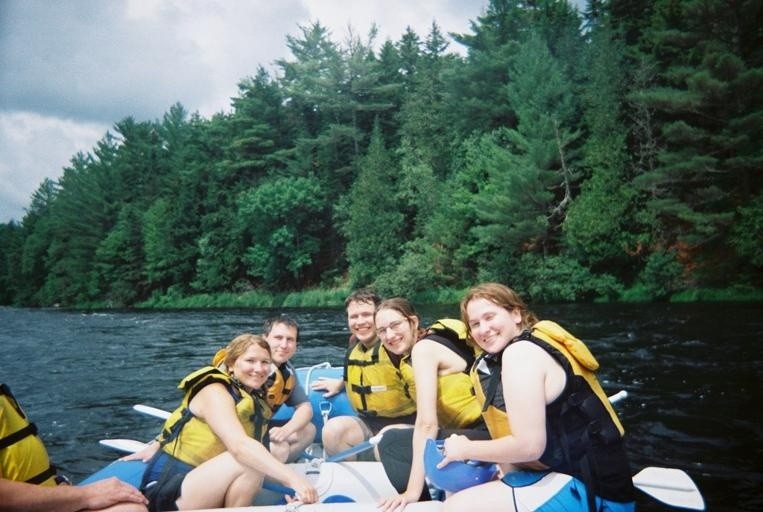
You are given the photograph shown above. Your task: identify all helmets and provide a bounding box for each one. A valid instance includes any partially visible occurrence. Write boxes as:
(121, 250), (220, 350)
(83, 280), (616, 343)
(423, 438), (500, 494)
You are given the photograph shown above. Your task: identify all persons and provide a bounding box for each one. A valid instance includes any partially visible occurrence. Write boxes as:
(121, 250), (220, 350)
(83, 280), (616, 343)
(371, 296), (484, 512)
(116, 315), (316, 466)
(137, 333), (320, 512)
(435, 282), (636, 512)
(306, 289), (416, 462)
(1, 381), (151, 512)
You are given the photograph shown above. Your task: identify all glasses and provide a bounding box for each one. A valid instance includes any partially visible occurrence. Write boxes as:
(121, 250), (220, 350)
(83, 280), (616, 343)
(374, 317), (408, 337)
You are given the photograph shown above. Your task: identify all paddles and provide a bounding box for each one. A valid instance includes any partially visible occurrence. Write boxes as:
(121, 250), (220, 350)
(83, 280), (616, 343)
(133, 404), (312, 460)
(633, 467), (705, 511)
(99, 438), (299, 497)
